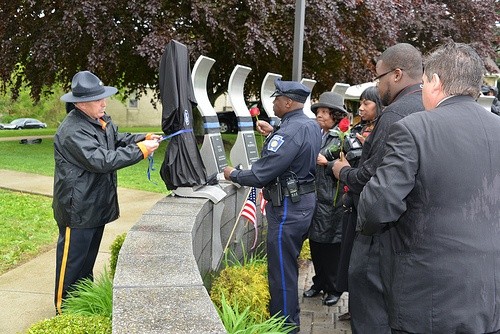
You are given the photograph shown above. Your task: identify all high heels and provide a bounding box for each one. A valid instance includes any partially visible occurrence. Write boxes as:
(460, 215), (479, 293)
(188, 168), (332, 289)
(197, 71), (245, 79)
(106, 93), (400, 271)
(304, 287), (326, 297)
(325, 292), (343, 306)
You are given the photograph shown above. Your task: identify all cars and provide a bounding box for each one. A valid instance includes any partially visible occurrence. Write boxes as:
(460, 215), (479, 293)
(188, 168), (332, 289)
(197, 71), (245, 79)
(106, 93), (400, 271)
(0, 117), (47, 130)
(216, 111), (239, 133)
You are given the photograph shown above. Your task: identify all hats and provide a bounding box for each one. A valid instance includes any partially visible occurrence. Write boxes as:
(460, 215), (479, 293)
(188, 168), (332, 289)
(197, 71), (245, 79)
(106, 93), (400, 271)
(60, 71), (118, 102)
(270, 77), (310, 103)
(311, 92), (349, 116)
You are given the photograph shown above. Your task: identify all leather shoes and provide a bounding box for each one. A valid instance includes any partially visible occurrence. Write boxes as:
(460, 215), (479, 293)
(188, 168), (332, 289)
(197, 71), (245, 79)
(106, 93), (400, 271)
(338, 313), (351, 320)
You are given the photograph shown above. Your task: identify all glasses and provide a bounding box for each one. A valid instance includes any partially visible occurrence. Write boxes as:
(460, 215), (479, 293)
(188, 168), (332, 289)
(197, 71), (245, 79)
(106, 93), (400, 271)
(373, 67), (402, 83)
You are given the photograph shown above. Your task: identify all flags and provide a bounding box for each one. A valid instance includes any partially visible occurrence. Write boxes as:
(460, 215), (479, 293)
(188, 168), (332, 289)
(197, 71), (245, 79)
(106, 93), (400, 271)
(259, 189), (268, 216)
(241, 187), (258, 249)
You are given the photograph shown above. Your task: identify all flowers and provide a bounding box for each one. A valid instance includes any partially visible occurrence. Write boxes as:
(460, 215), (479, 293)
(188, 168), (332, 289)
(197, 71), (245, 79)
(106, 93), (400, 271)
(327, 117), (351, 207)
(250, 106), (265, 151)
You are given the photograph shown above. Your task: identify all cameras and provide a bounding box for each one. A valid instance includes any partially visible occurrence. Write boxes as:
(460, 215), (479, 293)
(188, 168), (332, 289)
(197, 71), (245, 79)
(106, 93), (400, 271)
(325, 133), (363, 162)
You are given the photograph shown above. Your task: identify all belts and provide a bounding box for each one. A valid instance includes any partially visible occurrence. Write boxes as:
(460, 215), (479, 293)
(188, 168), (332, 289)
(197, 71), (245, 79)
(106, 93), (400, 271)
(262, 183), (315, 200)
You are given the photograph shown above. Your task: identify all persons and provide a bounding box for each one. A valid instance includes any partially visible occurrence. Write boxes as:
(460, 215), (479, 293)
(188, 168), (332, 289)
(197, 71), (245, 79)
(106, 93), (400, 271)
(303, 44), (425, 321)
(52, 71), (163, 314)
(357, 44), (500, 334)
(224, 80), (322, 334)
